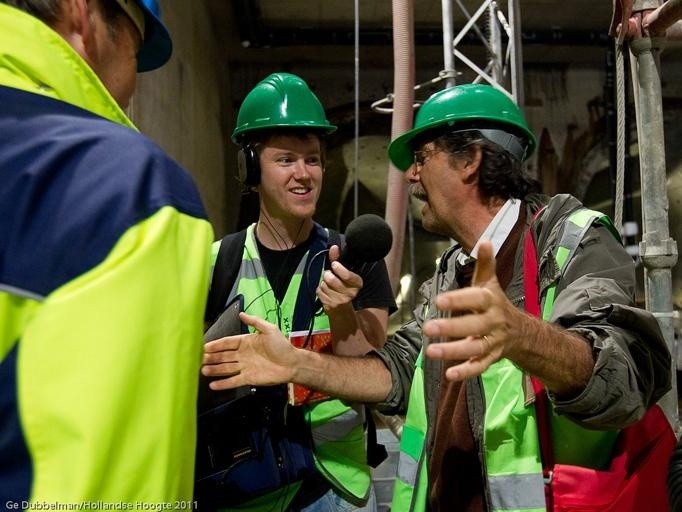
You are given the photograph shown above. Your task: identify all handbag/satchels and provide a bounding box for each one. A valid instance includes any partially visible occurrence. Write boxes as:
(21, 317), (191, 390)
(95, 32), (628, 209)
(543, 405), (676, 510)
(194, 391), (316, 510)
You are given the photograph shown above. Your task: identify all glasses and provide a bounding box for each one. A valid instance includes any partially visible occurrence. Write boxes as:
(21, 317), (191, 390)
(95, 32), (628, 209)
(413, 148), (464, 176)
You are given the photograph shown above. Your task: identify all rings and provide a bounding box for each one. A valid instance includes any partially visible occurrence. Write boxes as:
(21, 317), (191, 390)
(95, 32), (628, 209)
(481, 336), (490, 350)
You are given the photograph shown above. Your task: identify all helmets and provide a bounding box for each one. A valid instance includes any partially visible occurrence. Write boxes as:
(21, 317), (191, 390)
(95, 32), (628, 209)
(119, 1), (172, 72)
(232, 72), (337, 144)
(387, 82), (538, 174)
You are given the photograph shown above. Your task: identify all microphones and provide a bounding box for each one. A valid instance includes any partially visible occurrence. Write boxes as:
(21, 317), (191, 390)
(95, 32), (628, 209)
(310, 214), (392, 315)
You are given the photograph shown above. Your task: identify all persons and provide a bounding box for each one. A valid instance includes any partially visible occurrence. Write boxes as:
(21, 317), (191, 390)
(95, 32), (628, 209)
(0, 0), (215, 512)
(191, 73), (398, 512)
(200, 83), (673, 512)
